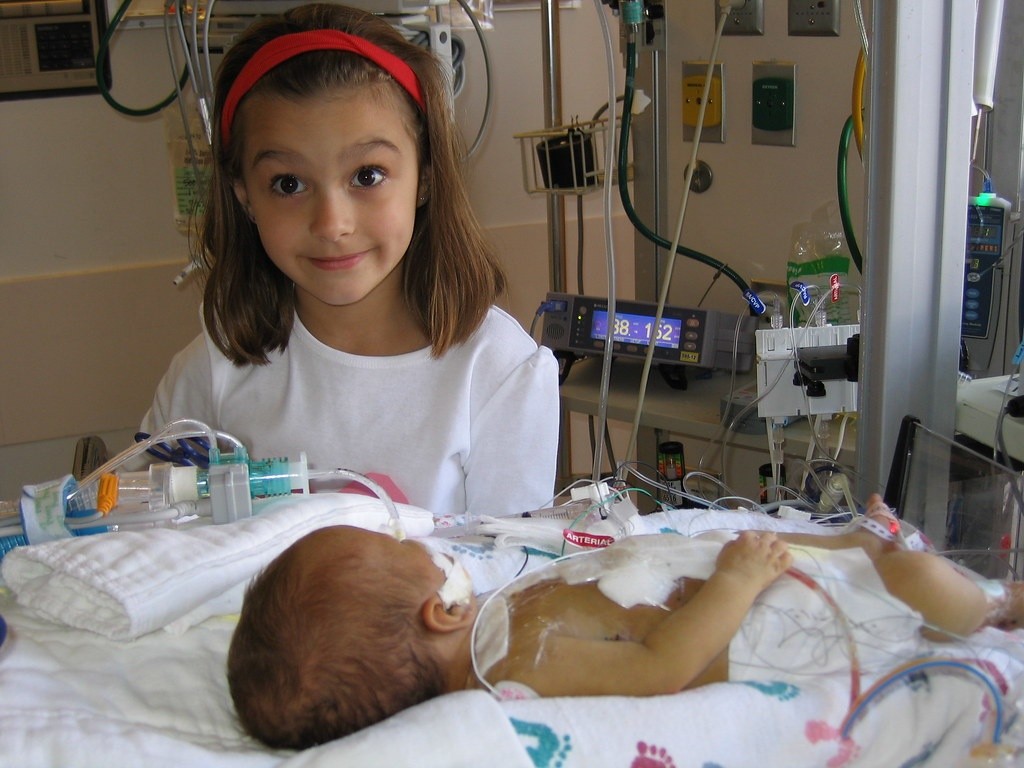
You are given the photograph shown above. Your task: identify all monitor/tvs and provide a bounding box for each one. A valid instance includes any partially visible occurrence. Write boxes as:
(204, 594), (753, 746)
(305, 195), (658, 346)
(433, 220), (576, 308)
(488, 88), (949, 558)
(0, 0), (112, 99)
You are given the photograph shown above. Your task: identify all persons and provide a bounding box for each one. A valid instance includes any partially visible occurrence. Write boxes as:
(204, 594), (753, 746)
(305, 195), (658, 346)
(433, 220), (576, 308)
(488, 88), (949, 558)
(226, 493), (1024, 751)
(112, 3), (558, 521)
